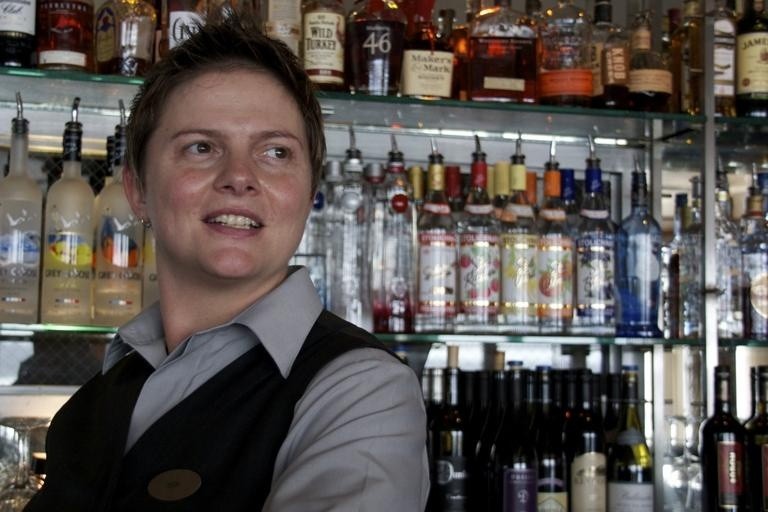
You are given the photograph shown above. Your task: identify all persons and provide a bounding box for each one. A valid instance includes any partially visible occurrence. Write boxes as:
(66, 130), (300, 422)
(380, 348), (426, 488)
(22, 22), (433, 512)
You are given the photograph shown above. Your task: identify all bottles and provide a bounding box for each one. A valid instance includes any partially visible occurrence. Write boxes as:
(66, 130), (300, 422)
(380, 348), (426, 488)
(0, 0), (767, 118)
(40, 122), (95, 328)
(288, 149), (767, 346)
(421, 345), (656, 511)
(95, 124), (146, 332)
(699, 363), (749, 512)
(1, 115), (41, 324)
(742, 364), (767, 512)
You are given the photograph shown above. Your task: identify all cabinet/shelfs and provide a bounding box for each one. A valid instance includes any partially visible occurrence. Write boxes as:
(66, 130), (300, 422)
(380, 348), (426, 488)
(0, 0), (768, 512)
